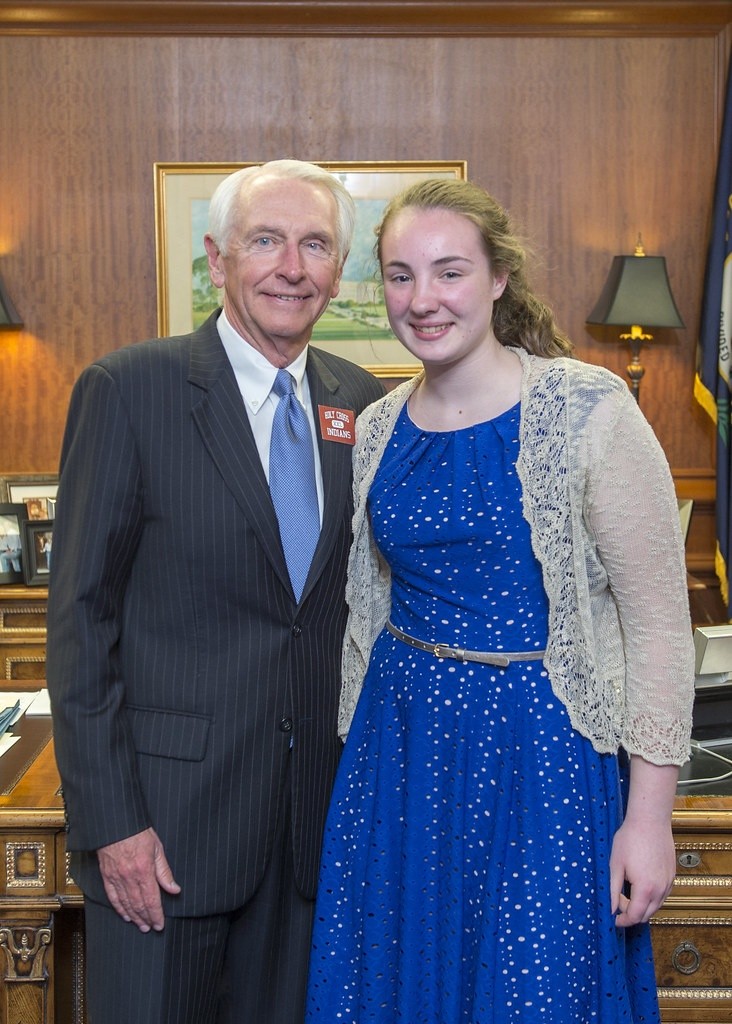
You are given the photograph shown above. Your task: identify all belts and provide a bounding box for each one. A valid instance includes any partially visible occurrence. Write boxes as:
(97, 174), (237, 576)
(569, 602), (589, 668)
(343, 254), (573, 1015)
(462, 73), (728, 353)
(384, 618), (546, 666)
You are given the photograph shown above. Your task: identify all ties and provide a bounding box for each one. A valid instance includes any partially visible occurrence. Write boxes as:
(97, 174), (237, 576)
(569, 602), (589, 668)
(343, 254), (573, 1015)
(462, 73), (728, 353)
(269, 369), (320, 749)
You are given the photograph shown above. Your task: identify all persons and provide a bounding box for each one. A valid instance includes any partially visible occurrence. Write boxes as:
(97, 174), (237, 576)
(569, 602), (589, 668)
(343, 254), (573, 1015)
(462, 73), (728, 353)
(302, 179), (696, 1024)
(38, 509), (47, 520)
(46, 160), (389, 1024)
(39, 537), (52, 570)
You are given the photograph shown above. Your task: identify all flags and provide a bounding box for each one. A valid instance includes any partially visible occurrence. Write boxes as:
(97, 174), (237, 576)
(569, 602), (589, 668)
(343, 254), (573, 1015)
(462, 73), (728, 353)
(694, 56), (732, 626)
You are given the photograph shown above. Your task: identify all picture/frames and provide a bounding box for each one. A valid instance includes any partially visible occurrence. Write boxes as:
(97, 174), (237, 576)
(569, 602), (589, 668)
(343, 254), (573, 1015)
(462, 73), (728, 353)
(0, 503), (30, 587)
(150, 158), (467, 379)
(19, 519), (53, 588)
(0, 471), (60, 521)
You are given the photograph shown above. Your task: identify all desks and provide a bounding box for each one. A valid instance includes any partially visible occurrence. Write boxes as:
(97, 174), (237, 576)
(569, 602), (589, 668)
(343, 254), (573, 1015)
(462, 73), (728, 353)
(0, 589), (51, 680)
(0, 679), (732, 1024)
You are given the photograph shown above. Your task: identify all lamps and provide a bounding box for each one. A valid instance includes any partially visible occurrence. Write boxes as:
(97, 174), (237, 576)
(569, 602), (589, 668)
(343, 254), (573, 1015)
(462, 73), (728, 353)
(584, 230), (687, 405)
